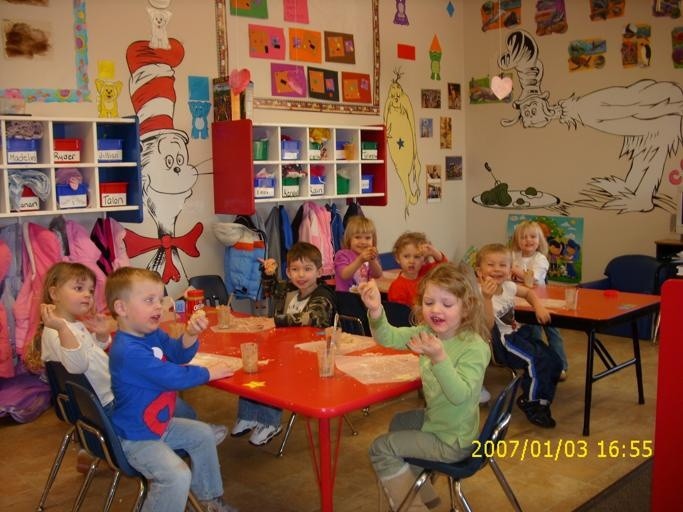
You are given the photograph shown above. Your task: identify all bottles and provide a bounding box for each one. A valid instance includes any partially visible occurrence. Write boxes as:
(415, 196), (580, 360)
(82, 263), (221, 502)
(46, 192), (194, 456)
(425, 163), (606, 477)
(187, 288), (206, 322)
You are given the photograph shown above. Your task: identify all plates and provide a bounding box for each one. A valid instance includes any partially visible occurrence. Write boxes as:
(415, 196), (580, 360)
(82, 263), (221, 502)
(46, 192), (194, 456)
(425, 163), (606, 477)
(472, 186), (560, 210)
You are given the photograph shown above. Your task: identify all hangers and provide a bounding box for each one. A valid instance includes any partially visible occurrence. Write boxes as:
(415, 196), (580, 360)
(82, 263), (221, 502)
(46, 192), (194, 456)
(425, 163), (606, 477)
(272, 195), (358, 214)
(15, 209), (107, 225)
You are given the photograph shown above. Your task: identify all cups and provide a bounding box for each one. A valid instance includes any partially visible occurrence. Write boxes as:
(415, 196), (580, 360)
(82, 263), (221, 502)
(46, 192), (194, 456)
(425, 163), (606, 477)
(317, 344), (337, 375)
(241, 342), (259, 375)
(171, 322), (189, 339)
(523, 270), (535, 287)
(217, 304), (231, 331)
(566, 289), (579, 309)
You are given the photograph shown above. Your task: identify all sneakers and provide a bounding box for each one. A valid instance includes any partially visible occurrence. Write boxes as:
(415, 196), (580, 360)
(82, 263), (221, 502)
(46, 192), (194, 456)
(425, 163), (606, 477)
(75, 448), (100, 475)
(248, 421), (283, 446)
(559, 370), (567, 382)
(198, 496), (240, 512)
(230, 417), (259, 438)
(516, 393), (556, 429)
(208, 424), (228, 448)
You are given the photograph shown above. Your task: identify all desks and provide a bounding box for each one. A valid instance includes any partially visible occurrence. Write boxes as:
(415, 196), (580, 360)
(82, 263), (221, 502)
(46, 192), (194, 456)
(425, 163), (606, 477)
(104, 308), (424, 511)
(368, 265), (662, 437)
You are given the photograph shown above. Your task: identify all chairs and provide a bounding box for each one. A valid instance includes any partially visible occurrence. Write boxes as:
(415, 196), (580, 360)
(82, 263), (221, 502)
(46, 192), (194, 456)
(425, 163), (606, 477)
(332, 289), (371, 337)
(273, 308), (359, 459)
(576, 253), (660, 343)
(477, 313), (529, 380)
(380, 300), (414, 328)
(392, 374), (527, 512)
(186, 273), (234, 313)
(31, 360), (122, 511)
(63, 378), (208, 511)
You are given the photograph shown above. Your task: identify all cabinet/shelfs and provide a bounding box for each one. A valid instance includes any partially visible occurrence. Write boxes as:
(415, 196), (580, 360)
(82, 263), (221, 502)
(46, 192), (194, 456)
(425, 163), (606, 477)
(209, 120), (390, 217)
(649, 237), (683, 342)
(0, 112), (146, 227)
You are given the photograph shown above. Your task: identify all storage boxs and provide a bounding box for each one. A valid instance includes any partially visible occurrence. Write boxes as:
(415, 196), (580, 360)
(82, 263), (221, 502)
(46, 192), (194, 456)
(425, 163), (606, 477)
(4, 136), (129, 214)
(251, 138), (379, 201)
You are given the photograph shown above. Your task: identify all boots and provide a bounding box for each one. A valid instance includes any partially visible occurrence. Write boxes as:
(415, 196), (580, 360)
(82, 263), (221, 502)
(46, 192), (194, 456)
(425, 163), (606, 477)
(409, 464), (443, 511)
(375, 462), (430, 512)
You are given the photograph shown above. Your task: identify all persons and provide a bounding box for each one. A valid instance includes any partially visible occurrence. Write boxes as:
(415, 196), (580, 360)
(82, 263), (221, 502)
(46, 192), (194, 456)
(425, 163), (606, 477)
(334, 216), (383, 293)
(384, 230), (452, 306)
(104, 268), (230, 511)
(508, 218), (570, 383)
(231, 242), (334, 445)
(470, 244), (561, 430)
(27, 261), (229, 447)
(355, 264), (495, 510)
